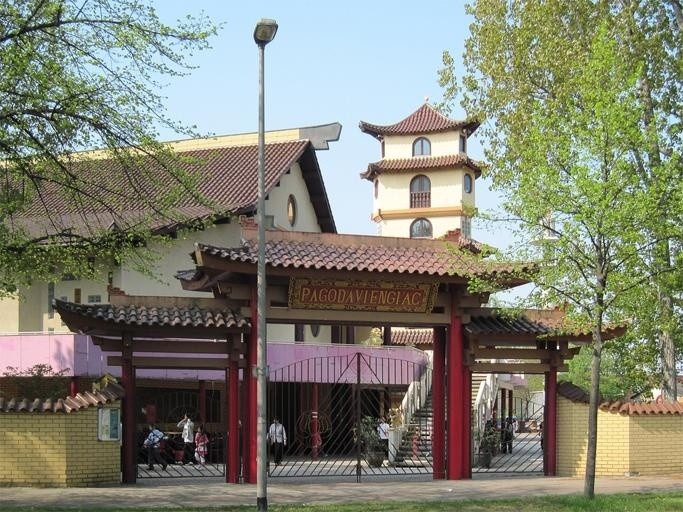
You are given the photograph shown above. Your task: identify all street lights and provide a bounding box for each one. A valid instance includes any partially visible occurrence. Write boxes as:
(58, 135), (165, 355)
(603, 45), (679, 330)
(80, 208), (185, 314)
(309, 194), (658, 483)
(254, 17), (278, 512)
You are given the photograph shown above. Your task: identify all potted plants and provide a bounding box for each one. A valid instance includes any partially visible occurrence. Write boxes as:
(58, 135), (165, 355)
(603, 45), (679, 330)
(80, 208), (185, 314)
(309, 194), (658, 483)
(352, 416), (390, 468)
(473, 427), (497, 468)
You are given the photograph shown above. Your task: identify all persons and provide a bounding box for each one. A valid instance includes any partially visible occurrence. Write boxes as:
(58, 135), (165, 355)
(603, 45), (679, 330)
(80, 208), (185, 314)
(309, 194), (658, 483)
(266, 416), (286, 467)
(143, 423), (167, 470)
(375, 416), (389, 458)
(192, 424), (208, 468)
(174, 411), (195, 466)
(501, 417), (514, 454)
